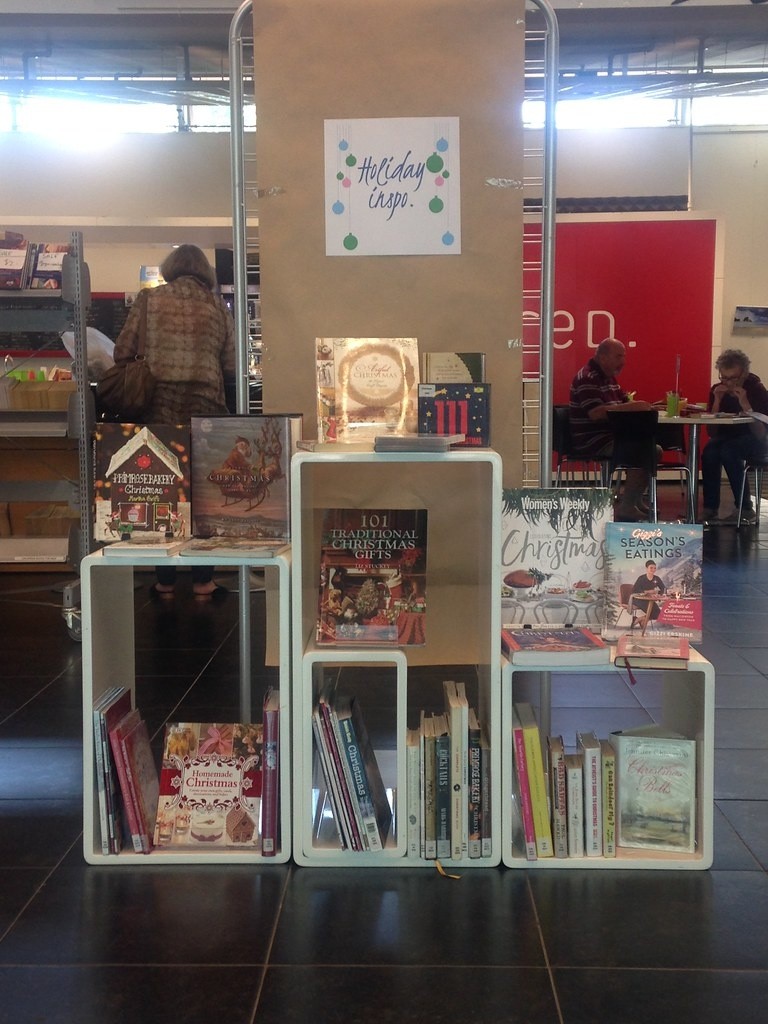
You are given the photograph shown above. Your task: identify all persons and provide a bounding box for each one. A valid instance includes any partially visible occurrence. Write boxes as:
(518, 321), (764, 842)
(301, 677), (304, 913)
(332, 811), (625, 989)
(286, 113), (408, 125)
(113, 244), (237, 595)
(630, 560), (667, 629)
(697, 348), (768, 523)
(565, 338), (664, 523)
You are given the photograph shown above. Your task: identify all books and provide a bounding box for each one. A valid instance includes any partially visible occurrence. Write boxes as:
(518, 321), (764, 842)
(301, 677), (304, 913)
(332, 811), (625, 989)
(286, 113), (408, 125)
(601, 520), (705, 643)
(261, 693), (280, 857)
(93, 687), (160, 854)
(314, 507), (429, 646)
(501, 627), (610, 667)
(512, 701), (697, 860)
(614, 634), (690, 671)
(180, 411), (304, 559)
(311, 677), (392, 853)
(503, 487), (614, 633)
(295, 439), (375, 452)
(152, 721), (262, 847)
(405, 681), (492, 860)
(87, 421), (193, 556)
(0, 231), (69, 290)
(314, 336), (491, 453)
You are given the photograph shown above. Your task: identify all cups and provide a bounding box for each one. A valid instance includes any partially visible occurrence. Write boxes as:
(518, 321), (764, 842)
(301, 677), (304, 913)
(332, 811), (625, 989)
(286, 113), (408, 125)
(678, 398), (687, 416)
(667, 397), (677, 416)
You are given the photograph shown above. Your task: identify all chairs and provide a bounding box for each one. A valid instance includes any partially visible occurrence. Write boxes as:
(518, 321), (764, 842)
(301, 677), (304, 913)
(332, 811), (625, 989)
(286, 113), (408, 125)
(606, 410), (657, 524)
(738, 430), (768, 527)
(649, 409), (694, 526)
(551, 404), (606, 501)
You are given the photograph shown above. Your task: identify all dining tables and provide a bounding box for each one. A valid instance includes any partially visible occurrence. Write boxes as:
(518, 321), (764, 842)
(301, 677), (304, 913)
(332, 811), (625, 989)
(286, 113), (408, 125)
(650, 411), (758, 523)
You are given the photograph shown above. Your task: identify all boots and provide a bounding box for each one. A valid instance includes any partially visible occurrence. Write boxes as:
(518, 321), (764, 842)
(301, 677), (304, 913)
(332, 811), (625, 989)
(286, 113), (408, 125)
(612, 469), (652, 522)
(637, 482), (660, 515)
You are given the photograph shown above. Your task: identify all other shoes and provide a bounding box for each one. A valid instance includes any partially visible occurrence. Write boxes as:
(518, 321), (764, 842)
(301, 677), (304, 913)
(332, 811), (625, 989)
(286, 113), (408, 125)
(638, 622), (644, 629)
(630, 616), (638, 630)
(193, 580), (228, 597)
(148, 583), (174, 594)
(718, 507), (758, 526)
(696, 506), (719, 524)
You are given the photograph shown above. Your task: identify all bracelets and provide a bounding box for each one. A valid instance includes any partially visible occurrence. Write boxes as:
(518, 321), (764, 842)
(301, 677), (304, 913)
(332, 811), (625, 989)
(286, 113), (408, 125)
(747, 408), (754, 412)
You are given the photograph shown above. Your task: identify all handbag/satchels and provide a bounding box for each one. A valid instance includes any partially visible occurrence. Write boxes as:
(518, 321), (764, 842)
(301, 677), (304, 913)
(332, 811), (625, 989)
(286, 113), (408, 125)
(92, 354), (156, 423)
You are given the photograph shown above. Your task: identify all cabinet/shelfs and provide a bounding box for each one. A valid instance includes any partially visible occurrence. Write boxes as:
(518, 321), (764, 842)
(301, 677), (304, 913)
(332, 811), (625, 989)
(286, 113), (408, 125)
(292, 442), (503, 868)
(0, 230), (90, 627)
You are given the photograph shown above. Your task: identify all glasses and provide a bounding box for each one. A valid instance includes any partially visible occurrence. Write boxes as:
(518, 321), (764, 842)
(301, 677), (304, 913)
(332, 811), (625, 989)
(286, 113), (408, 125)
(717, 369), (745, 382)
